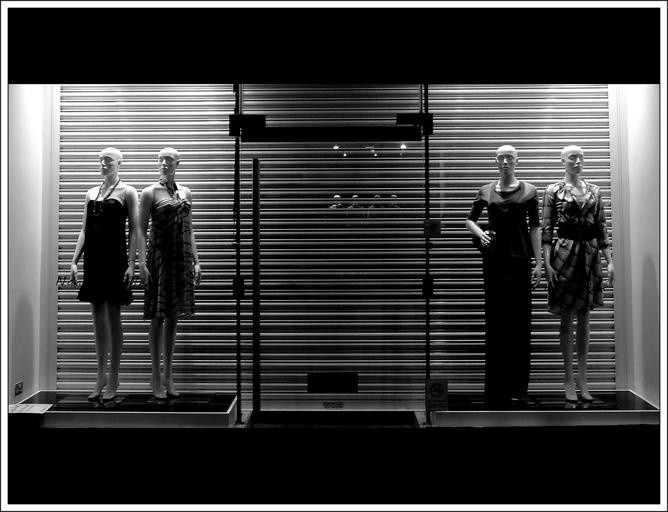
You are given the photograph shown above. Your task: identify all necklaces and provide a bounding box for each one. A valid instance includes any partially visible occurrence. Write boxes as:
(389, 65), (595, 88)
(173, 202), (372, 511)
(93, 178), (121, 216)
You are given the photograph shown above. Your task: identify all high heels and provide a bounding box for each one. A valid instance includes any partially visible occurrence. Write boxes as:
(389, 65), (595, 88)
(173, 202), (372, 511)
(88, 381), (178, 400)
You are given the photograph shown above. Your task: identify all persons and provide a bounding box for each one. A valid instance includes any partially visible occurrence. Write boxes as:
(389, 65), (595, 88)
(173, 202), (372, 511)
(467, 143), (543, 403)
(137, 146), (202, 399)
(69, 147), (141, 400)
(329, 194), (400, 220)
(542, 145), (615, 403)
